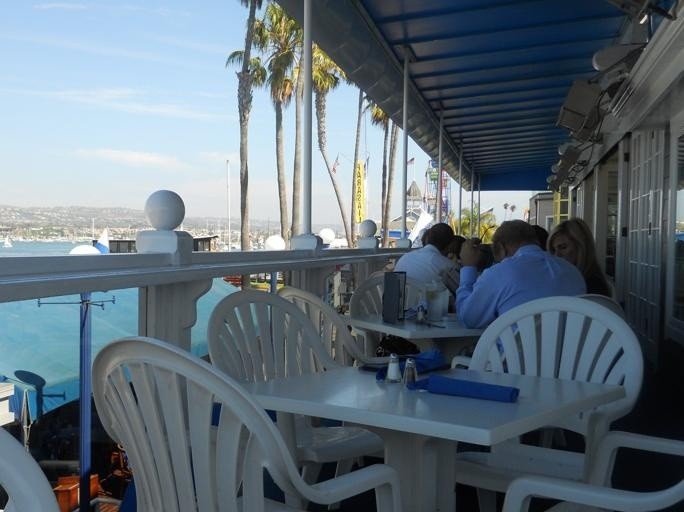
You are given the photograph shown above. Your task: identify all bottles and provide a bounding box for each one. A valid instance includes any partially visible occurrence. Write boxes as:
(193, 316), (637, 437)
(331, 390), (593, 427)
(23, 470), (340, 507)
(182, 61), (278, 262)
(386, 354), (417, 387)
(416, 305), (424, 321)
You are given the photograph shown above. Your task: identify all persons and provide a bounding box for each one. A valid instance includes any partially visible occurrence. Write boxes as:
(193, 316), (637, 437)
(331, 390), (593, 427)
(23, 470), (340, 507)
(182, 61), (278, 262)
(392, 218), (613, 372)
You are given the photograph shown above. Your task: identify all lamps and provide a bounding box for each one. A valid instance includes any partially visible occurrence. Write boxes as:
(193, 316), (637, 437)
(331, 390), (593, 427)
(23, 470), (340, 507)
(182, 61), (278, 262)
(547, 141), (587, 182)
(592, 40), (648, 71)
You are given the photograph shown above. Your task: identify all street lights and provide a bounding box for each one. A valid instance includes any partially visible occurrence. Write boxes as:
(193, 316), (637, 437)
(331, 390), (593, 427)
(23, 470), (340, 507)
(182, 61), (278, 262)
(68, 245), (102, 511)
(318, 227), (334, 302)
(263, 236), (285, 362)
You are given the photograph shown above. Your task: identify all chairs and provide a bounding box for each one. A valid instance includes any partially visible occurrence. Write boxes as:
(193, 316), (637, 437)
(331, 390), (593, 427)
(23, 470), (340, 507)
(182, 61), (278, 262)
(92, 336), (401, 511)
(503, 432), (683, 511)
(451, 295), (644, 509)
(206, 272), (444, 508)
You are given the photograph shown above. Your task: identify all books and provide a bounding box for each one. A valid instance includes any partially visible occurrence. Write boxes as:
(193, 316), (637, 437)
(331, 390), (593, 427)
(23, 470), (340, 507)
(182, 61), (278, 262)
(382, 271), (406, 324)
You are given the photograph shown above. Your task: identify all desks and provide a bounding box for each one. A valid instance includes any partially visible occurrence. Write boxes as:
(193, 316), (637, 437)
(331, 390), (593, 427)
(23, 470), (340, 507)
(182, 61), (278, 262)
(341, 312), (485, 354)
(239, 364), (625, 511)
